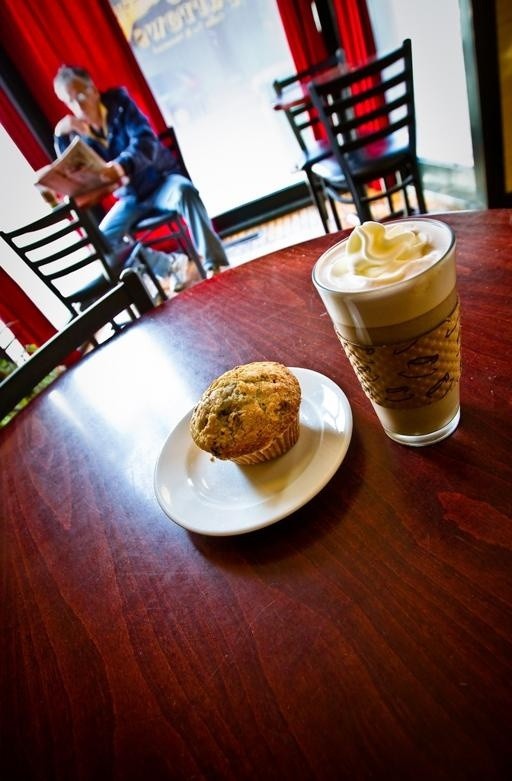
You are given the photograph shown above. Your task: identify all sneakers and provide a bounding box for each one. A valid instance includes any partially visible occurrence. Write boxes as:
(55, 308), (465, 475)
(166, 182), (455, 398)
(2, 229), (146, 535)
(168, 252), (188, 292)
(206, 265), (220, 280)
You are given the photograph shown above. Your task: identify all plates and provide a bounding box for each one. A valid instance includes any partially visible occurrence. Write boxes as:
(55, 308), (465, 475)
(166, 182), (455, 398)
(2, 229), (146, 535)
(153, 366), (353, 538)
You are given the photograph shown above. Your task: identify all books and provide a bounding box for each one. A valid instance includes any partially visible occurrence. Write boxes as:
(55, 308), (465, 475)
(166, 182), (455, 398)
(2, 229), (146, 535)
(31, 136), (112, 201)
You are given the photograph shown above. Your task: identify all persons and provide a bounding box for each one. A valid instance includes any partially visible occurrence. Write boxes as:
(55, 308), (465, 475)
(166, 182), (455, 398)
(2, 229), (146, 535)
(39, 63), (231, 296)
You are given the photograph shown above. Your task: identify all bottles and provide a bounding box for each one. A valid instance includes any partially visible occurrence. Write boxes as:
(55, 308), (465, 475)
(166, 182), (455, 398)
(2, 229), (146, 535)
(316, 217), (464, 449)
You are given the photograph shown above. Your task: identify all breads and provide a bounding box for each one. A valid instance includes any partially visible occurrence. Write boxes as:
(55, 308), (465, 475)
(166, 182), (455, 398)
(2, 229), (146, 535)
(189, 362), (302, 466)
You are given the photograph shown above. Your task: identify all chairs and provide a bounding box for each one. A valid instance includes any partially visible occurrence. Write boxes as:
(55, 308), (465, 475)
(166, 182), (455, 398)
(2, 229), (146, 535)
(271, 35), (431, 235)
